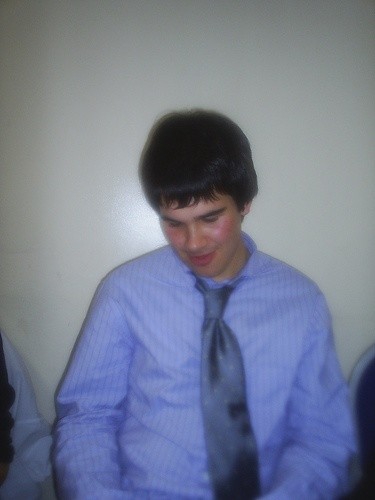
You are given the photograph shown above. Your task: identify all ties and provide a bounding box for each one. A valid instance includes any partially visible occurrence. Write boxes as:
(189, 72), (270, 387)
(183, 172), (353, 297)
(195, 281), (260, 500)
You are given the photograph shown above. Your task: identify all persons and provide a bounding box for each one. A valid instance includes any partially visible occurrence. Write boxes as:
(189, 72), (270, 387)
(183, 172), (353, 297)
(53, 109), (362, 500)
(1, 334), (53, 499)
